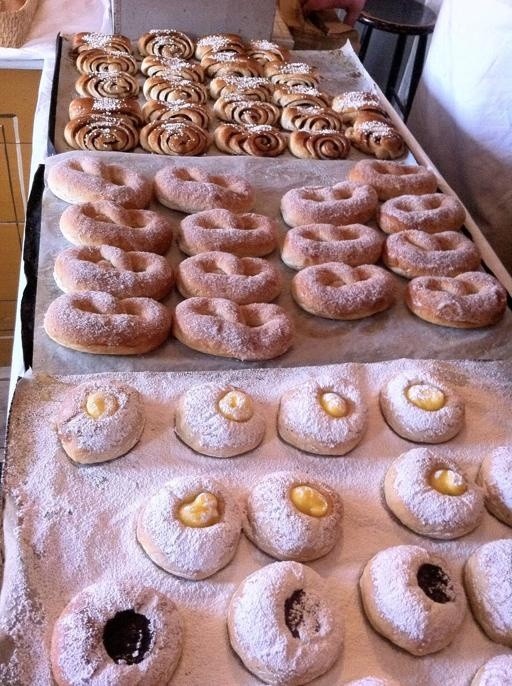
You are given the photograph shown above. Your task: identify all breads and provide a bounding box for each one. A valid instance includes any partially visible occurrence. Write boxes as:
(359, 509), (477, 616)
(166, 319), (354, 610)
(228, 559), (346, 685)
(403, 271), (504, 328)
(463, 540), (511, 647)
(49, 582), (186, 686)
(135, 474), (242, 581)
(345, 675), (389, 686)
(250, 471), (342, 563)
(473, 446), (512, 527)
(173, 300), (290, 359)
(175, 382), (265, 457)
(52, 378), (145, 465)
(44, 292), (168, 353)
(471, 654), (512, 686)
(359, 544), (466, 656)
(380, 370), (464, 443)
(65, 30), (404, 157)
(290, 260), (396, 320)
(385, 448), (485, 539)
(277, 376), (367, 456)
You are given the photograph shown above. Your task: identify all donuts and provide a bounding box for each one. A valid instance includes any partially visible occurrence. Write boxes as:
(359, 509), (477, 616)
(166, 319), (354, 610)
(47, 158), (482, 297)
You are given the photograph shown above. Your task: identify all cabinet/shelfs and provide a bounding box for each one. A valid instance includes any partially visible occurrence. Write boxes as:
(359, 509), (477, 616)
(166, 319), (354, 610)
(0, 0), (114, 367)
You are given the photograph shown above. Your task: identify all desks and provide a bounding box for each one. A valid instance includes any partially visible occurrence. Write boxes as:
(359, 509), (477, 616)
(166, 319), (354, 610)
(2, 31), (511, 686)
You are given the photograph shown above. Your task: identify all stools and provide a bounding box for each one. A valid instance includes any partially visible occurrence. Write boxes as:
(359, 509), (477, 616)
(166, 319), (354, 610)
(357, 1), (437, 122)
